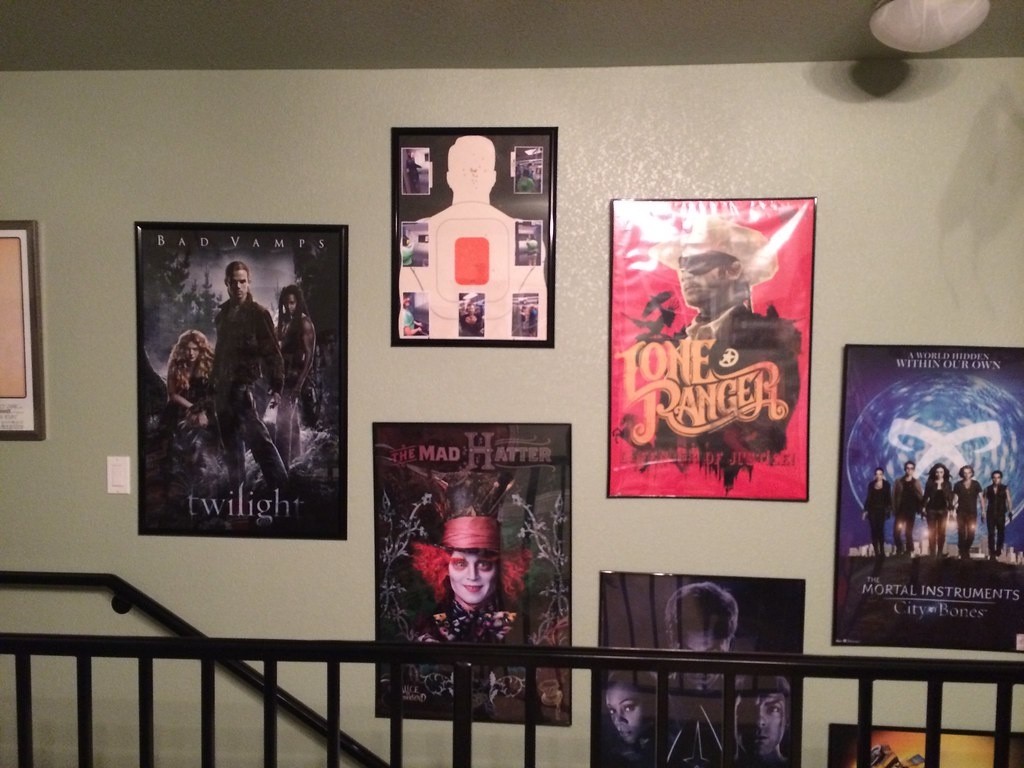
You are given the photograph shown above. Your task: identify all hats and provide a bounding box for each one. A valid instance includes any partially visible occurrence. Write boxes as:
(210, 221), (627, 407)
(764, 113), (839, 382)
(648, 217), (779, 287)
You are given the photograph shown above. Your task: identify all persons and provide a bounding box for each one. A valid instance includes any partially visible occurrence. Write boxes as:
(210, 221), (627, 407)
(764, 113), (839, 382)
(525, 235), (539, 266)
(162, 329), (215, 439)
(981, 470), (1013, 561)
(461, 299), (485, 337)
(951, 465), (986, 559)
(405, 149), (423, 193)
(645, 581), (742, 768)
(403, 294), (423, 336)
(891, 460), (923, 556)
(210, 260), (299, 508)
(606, 668), (683, 768)
(733, 673), (790, 768)
(402, 235), (414, 267)
(521, 304), (537, 321)
(919, 463), (954, 556)
(651, 214), (801, 453)
(273, 284), (315, 470)
(861, 465), (893, 560)
(405, 516), (534, 698)
(516, 163), (536, 193)
